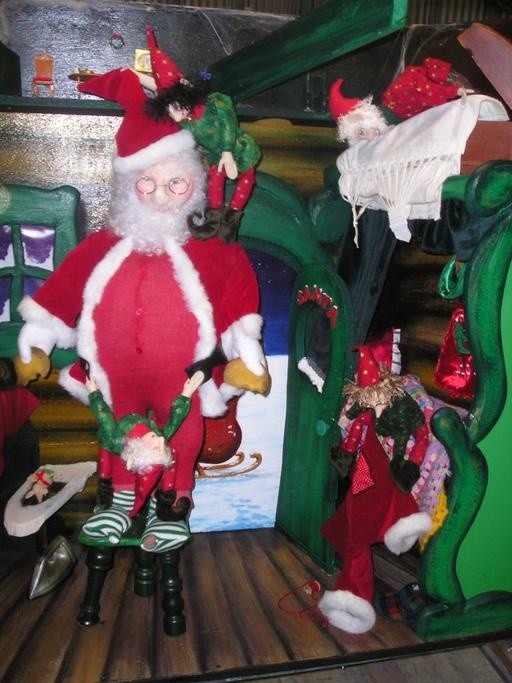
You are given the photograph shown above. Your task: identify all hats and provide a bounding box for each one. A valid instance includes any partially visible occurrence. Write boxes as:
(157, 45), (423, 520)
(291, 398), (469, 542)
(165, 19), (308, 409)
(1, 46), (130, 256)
(349, 345), (402, 405)
(317, 489), (380, 638)
(324, 78), (371, 123)
(78, 69), (196, 173)
(361, 416), (433, 557)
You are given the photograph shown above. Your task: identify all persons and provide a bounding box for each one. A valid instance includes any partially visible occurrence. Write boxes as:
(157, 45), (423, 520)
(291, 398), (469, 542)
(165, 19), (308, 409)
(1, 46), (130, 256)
(16, 27), (269, 556)
(329, 54), (474, 148)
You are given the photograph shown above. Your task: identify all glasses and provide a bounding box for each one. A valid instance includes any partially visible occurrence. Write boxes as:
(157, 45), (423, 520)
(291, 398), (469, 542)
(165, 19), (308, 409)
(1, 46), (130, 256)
(127, 176), (193, 196)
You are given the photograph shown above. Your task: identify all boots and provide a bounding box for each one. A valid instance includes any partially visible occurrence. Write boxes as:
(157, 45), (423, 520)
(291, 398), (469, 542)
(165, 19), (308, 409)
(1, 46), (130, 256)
(219, 206), (243, 245)
(184, 206), (224, 240)
